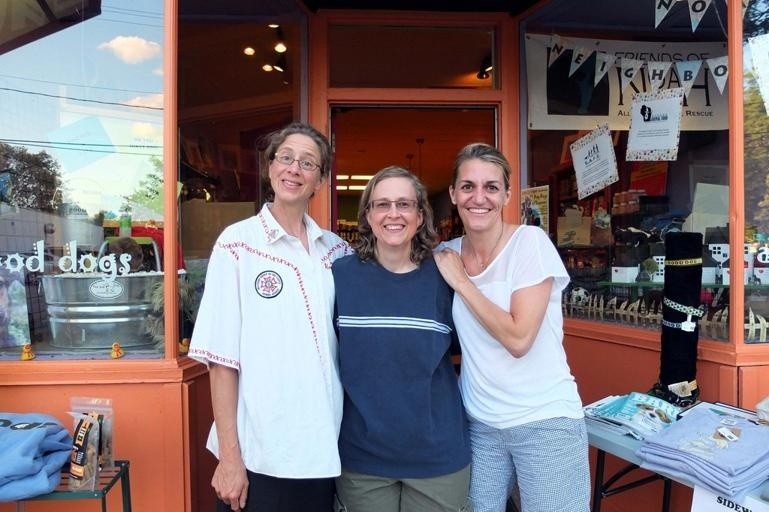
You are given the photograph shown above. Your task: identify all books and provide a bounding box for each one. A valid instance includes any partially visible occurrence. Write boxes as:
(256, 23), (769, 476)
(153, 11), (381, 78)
(576, 390), (680, 441)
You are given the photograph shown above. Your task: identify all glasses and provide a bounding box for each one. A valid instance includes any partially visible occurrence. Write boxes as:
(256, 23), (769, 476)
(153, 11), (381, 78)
(365, 199), (420, 214)
(270, 153), (325, 176)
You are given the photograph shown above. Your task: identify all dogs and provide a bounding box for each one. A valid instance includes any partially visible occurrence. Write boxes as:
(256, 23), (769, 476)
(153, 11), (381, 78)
(625, 403), (673, 437)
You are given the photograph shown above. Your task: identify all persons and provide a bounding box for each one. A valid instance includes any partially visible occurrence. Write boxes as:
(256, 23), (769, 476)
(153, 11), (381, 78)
(322, 164), (480, 511)
(422, 140), (593, 511)
(183, 121), (369, 511)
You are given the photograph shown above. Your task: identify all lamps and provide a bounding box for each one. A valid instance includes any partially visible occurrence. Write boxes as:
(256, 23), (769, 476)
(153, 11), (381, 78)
(272, 55), (289, 74)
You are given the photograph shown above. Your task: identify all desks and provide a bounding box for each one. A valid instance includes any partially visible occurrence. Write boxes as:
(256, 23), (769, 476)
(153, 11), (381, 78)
(584, 400), (768, 511)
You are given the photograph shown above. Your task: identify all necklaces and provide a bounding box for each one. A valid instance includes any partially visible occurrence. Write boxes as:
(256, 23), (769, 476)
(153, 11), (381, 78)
(468, 221), (505, 274)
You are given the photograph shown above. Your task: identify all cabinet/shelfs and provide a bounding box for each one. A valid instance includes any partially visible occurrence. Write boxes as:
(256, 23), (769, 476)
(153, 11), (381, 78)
(548, 144), (672, 270)
(17, 460), (132, 512)
